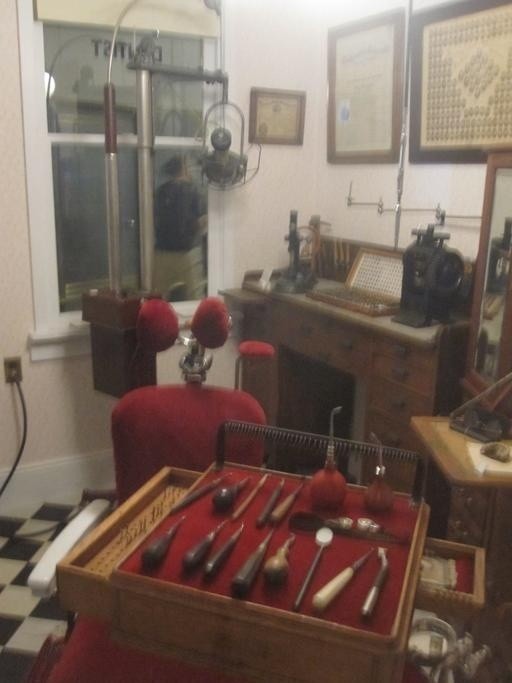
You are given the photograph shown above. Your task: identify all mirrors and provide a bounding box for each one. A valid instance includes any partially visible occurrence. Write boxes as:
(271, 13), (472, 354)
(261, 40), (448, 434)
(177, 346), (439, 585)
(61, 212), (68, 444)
(459, 152), (512, 418)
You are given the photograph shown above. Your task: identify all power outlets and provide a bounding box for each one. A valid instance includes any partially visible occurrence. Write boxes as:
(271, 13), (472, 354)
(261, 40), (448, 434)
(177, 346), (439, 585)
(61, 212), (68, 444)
(4, 357), (22, 383)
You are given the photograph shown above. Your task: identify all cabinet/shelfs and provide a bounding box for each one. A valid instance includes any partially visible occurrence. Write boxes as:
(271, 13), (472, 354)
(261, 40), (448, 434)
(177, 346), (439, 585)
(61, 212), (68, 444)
(218, 269), (472, 495)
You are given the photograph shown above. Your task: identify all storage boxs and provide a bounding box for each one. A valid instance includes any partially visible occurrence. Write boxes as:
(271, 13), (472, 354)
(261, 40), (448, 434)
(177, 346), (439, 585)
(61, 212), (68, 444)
(304, 247), (404, 317)
(55, 455), (487, 681)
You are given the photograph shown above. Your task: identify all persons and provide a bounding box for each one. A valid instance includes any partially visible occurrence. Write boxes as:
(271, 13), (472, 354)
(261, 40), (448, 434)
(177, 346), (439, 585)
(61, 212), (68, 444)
(154, 156), (207, 299)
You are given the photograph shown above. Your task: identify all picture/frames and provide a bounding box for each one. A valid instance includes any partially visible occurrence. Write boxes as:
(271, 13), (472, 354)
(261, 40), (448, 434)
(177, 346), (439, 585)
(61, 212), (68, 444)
(326, 4), (402, 163)
(248, 87), (306, 146)
(408, 2), (511, 164)
